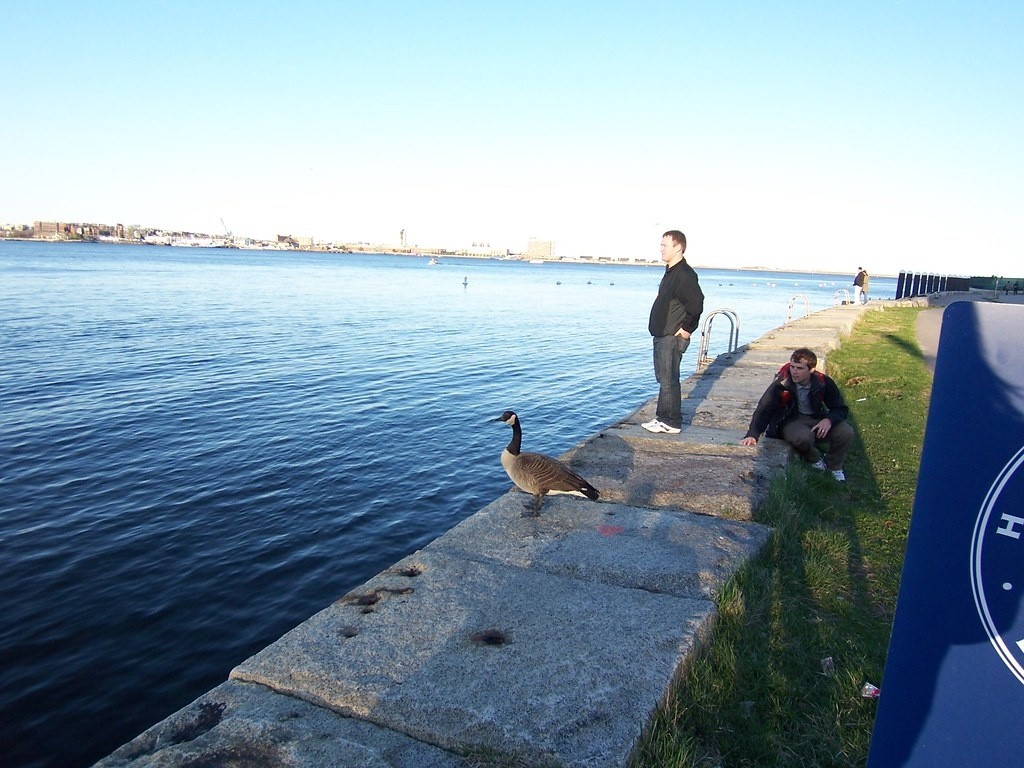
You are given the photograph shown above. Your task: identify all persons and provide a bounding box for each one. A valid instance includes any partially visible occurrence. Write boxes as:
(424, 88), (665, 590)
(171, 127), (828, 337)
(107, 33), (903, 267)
(740, 347), (853, 482)
(862, 270), (869, 303)
(648, 229), (705, 435)
(852, 266), (863, 305)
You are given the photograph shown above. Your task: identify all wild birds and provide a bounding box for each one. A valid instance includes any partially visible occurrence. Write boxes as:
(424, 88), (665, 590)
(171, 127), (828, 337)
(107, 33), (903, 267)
(494, 411), (602, 519)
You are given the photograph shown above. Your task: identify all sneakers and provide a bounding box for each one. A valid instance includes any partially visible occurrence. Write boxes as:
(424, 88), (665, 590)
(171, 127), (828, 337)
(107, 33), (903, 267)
(829, 469), (845, 481)
(646, 421), (682, 434)
(808, 455), (828, 471)
(641, 419), (660, 429)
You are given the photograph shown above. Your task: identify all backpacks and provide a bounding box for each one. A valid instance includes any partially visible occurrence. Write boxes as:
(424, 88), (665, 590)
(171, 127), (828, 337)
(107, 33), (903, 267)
(774, 362), (825, 406)
